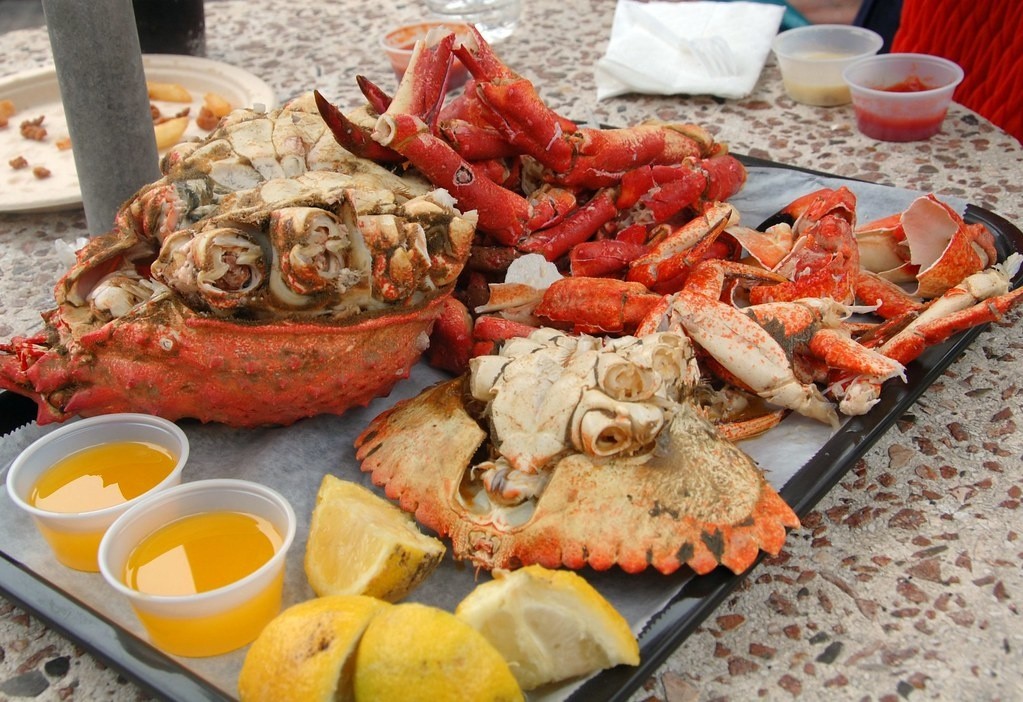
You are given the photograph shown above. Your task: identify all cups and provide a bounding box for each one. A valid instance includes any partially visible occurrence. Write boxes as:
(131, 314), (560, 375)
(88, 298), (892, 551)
(845, 52), (965, 143)
(100, 479), (296, 657)
(771, 23), (884, 106)
(4, 412), (189, 572)
(424, 1), (521, 45)
(379, 20), (469, 93)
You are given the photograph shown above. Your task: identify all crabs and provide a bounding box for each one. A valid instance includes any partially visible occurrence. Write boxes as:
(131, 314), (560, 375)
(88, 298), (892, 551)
(0, 20), (1020, 580)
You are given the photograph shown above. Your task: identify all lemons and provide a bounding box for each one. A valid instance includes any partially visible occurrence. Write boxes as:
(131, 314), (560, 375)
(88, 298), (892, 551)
(238, 472), (642, 701)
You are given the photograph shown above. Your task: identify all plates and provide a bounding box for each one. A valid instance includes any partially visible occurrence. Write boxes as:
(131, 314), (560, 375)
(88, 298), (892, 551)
(0, 54), (277, 219)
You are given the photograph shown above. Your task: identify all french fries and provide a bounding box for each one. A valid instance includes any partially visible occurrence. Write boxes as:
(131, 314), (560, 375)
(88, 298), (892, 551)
(145, 80), (230, 149)
